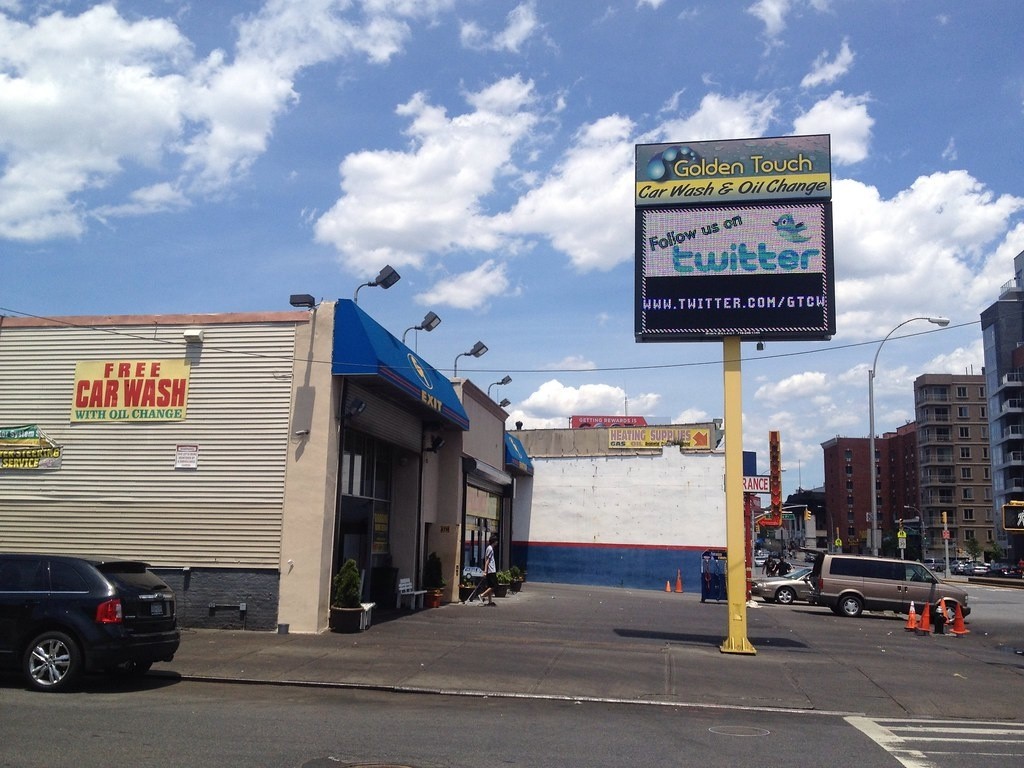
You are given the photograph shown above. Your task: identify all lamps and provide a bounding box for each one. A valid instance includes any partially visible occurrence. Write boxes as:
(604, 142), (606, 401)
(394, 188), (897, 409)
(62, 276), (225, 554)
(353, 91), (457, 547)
(341, 398), (367, 417)
(454, 340), (489, 377)
(403, 311), (441, 343)
(289, 294), (317, 309)
(353, 264), (401, 303)
(500, 398), (510, 407)
(425, 435), (446, 452)
(184, 330), (204, 342)
(488, 375), (512, 396)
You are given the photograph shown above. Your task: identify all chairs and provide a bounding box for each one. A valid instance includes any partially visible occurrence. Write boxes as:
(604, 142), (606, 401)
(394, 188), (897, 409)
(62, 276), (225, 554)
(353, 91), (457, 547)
(397, 578), (427, 610)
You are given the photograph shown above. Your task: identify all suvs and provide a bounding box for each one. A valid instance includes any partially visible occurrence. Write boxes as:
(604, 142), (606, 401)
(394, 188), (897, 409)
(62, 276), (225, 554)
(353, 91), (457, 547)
(809, 553), (973, 620)
(0, 552), (183, 690)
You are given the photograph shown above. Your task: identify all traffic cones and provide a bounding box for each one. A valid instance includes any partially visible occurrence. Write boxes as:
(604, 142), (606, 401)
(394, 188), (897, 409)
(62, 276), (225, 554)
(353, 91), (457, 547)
(938, 596), (952, 626)
(672, 569), (684, 593)
(950, 603), (976, 636)
(915, 600), (932, 632)
(666, 580), (672, 592)
(905, 599), (926, 632)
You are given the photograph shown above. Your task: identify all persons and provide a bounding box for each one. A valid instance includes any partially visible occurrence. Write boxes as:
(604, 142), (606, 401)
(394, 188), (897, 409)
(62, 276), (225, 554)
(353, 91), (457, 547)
(1017, 557), (1024, 570)
(478, 534), (498, 606)
(774, 556), (791, 577)
(762, 554), (776, 577)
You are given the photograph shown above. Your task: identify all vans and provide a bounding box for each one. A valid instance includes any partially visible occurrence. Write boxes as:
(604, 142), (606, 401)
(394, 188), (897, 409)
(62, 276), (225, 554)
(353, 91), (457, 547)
(923, 557), (949, 568)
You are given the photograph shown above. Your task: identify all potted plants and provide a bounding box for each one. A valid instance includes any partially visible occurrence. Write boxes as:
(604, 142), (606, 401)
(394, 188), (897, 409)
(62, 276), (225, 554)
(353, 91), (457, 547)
(423, 552), (447, 608)
(493, 569), (513, 597)
(330, 558), (364, 631)
(509, 566), (527, 591)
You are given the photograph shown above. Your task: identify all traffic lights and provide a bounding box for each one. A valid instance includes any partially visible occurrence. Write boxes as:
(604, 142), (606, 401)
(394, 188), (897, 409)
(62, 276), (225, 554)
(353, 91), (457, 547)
(1002, 505), (1024, 531)
(940, 511), (945, 523)
(804, 510), (813, 521)
(899, 519), (904, 531)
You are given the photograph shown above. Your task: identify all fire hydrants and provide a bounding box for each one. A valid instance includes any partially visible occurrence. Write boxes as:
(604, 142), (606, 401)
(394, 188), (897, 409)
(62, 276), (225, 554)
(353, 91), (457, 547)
(932, 606), (948, 635)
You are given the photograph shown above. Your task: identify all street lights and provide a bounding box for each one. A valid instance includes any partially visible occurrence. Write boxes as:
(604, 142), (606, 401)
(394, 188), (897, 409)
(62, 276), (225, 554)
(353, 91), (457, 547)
(751, 468), (787, 577)
(817, 505), (833, 554)
(903, 506), (926, 564)
(866, 316), (951, 555)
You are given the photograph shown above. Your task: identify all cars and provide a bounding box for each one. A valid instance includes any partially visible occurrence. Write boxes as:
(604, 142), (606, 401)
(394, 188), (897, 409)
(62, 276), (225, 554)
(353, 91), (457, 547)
(985, 563), (1023, 576)
(951, 559), (965, 575)
(753, 547), (780, 567)
(964, 560), (993, 577)
(750, 567), (823, 604)
(804, 554), (816, 562)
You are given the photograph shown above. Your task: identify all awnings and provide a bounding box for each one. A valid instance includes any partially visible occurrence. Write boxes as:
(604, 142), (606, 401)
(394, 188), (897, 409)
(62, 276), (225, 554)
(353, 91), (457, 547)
(504, 431), (535, 477)
(331, 299), (469, 432)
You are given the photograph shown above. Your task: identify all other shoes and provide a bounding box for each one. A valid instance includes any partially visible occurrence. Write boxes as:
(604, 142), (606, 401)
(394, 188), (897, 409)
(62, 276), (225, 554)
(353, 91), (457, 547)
(489, 602), (496, 606)
(478, 594), (484, 604)
